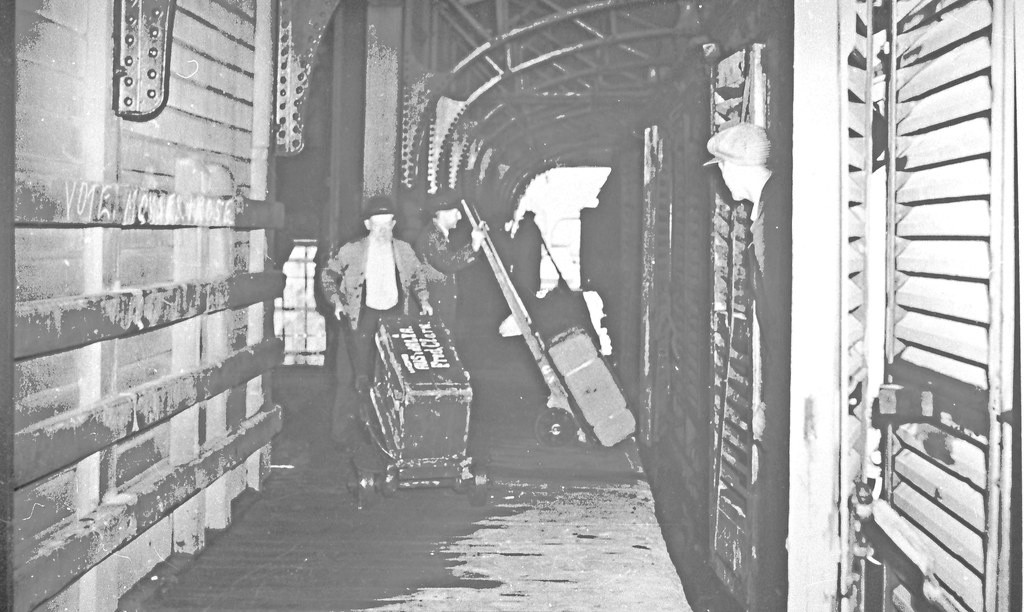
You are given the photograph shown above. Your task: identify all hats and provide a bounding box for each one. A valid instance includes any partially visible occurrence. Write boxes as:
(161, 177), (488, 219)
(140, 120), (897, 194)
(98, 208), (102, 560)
(363, 193), (397, 218)
(421, 187), (461, 218)
(702, 122), (770, 168)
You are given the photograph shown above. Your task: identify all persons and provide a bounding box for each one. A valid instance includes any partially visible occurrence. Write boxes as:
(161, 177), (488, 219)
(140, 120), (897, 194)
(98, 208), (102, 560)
(318, 187), (544, 487)
(703, 124), (792, 456)
